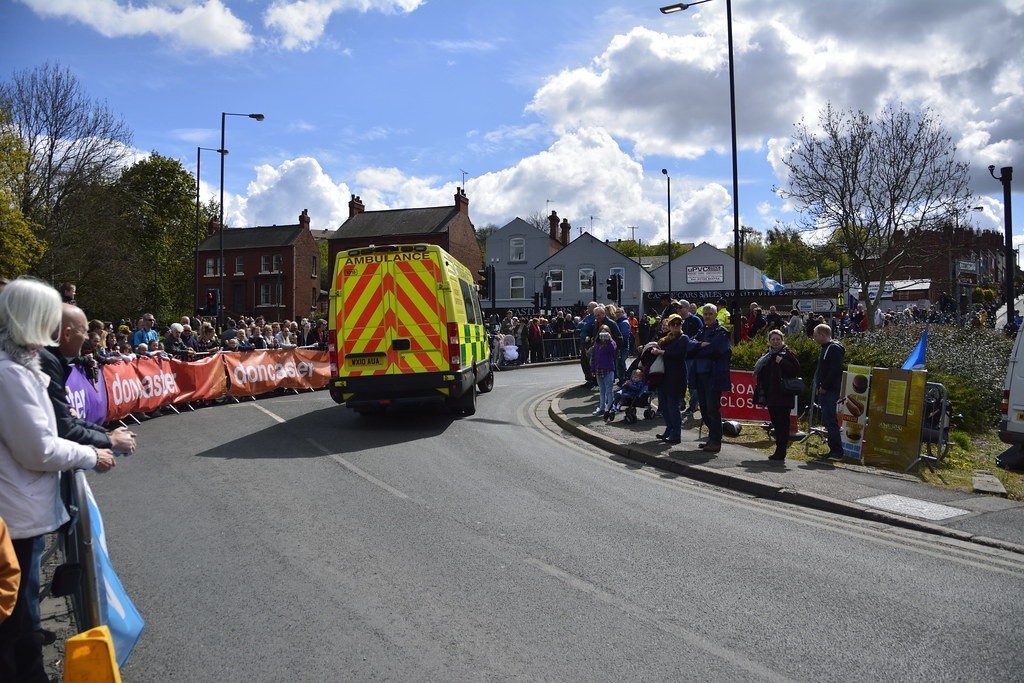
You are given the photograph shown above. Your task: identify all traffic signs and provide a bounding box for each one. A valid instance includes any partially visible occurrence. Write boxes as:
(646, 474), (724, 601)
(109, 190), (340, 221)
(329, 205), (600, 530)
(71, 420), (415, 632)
(793, 299), (837, 312)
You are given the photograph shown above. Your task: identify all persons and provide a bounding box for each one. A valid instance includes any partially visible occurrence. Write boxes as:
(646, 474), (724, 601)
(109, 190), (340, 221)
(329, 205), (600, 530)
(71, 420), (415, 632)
(480, 293), (868, 460)
(0, 274), (328, 683)
(875, 304), (1022, 340)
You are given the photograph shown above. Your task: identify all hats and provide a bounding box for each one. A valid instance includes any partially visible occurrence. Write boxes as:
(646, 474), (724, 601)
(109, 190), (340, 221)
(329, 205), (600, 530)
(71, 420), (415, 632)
(673, 299), (689, 307)
(668, 313), (683, 325)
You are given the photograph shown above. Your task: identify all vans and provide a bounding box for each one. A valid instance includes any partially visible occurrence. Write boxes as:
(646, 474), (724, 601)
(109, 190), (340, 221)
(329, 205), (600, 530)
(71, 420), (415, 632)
(999, 318), (1024, 446)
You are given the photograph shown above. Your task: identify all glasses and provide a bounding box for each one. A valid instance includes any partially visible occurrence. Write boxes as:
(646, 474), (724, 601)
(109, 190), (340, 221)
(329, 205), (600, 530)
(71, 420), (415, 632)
(671, 321), (682, 327)
(676, 306), (685, 309)
(184, 330), (191, 332)
(147, 319), (155, 321)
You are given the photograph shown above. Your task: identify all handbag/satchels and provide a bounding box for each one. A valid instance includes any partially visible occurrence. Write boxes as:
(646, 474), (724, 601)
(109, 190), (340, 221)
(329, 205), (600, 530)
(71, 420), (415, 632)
(778, 376), (805, 393)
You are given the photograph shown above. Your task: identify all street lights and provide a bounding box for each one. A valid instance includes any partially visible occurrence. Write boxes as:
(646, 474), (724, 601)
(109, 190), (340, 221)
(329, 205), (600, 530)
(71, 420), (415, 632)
(217, 112), (266, 332)
(194, 147), (230, 316)
(661, 168), (671, 297)
(836, 245), (848, 338)
(955, 206), (983, 329)
(661, 0), (741, 347)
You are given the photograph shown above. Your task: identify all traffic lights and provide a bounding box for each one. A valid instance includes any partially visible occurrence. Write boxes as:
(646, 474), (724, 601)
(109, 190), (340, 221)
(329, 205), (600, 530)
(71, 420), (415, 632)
(606, 275), (617, 300)
(531, 292), (539, 313)
(207, 289), (220, 317)
(478, 266), (491, 301)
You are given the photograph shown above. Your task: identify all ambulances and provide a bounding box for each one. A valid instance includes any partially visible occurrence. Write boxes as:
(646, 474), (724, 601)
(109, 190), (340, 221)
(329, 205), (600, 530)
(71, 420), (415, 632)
(327, 242), (500, 419)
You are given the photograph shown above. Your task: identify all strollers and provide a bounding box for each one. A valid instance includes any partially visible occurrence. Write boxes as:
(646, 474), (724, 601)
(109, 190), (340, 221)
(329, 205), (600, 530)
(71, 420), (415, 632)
(604, 342), (656, 424)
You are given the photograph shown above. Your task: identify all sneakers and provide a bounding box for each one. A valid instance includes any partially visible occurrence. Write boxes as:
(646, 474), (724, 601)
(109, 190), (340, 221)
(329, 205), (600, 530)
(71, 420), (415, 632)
(818, 451), (843, 461)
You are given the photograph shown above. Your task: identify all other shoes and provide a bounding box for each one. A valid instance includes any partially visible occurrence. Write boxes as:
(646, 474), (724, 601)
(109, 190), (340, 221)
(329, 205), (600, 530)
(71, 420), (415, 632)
(662, 436), (681, 443)
(593, 410), (605, 415)
(698, 440), (712, 448)
(703, 443), (721, 451)
(656, 433), (668, 439)
